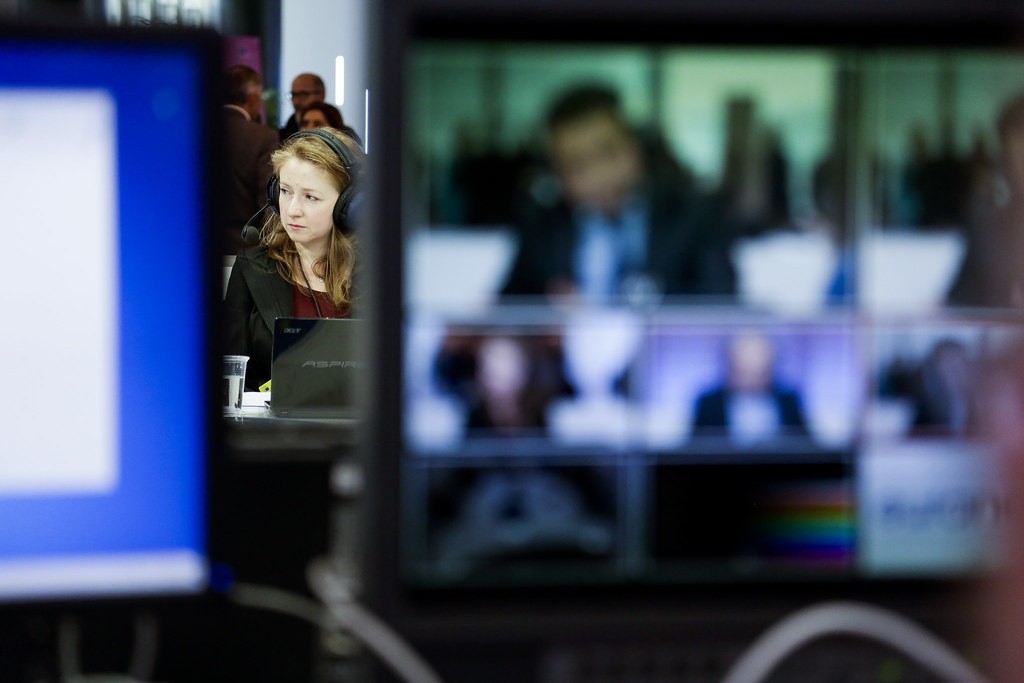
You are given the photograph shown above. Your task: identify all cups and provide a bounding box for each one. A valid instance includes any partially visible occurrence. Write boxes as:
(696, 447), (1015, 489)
(225, 356), (251, 414)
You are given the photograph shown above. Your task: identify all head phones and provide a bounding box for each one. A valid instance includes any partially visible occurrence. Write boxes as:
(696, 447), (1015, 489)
(267, 126), (364, 238)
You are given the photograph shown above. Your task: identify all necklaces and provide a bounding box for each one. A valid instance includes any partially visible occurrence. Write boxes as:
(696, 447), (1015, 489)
(296, 255), (322, 318)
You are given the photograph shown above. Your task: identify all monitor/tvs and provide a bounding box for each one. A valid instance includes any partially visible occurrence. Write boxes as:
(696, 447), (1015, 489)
(359, 2), (1023, 683)
(0, 10), (234, 625)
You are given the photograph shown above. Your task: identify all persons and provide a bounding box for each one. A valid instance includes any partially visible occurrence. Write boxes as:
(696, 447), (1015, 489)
(279, 74), (362, 145)
(431, 81), (1024, 309)
(298, 100), (344, 131)
(908, 337), (986, 442)
(461, 340), (552, 442)
(224, 65), (279, 255)
(225, 127), (368, 392)
(689, 335), (813, 440)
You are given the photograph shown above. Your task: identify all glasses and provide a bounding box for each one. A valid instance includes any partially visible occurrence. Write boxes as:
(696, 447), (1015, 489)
(299, 119), (323, 128)
(290, 91), (317, 98)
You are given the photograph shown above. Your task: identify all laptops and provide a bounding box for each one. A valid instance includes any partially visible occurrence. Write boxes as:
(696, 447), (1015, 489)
(265, 315), (363, 420)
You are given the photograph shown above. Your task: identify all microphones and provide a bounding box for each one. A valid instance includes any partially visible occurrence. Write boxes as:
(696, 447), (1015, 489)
(241, 203), (268, 244)
